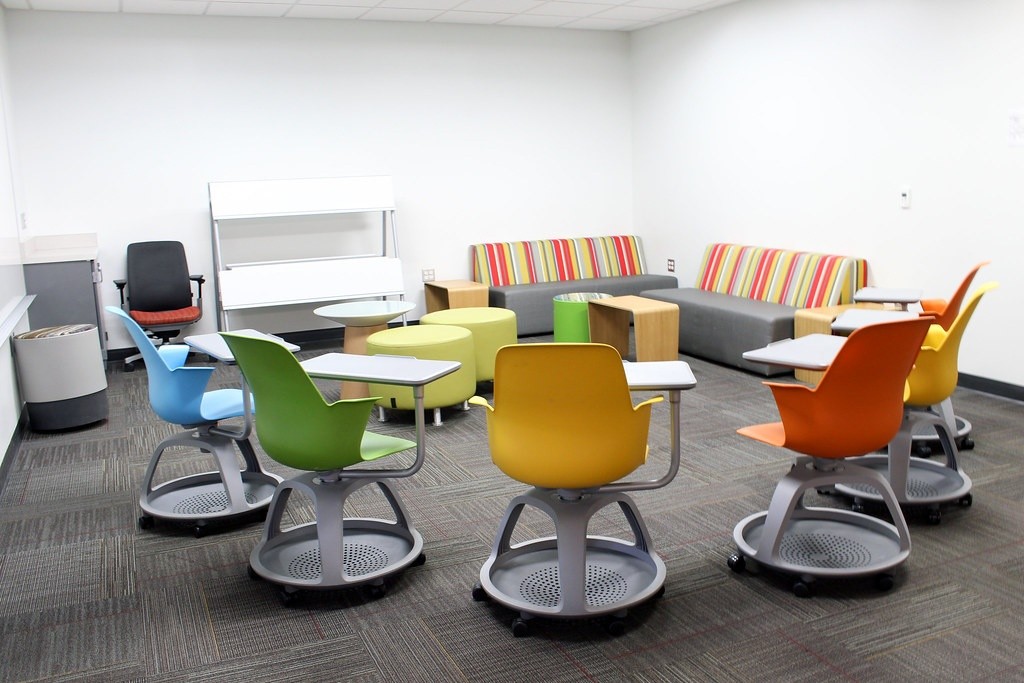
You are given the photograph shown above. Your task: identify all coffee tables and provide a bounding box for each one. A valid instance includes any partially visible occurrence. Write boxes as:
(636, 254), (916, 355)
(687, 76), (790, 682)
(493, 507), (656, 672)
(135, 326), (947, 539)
(793, 301), (885, 384)
(588, 294), (679, 362)
(424, 278), (489, 313)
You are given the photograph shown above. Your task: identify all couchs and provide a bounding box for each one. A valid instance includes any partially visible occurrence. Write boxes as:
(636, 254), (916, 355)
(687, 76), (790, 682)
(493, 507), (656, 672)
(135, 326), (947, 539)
(471, 234), (678, 337)
(640, 241), (868, 375)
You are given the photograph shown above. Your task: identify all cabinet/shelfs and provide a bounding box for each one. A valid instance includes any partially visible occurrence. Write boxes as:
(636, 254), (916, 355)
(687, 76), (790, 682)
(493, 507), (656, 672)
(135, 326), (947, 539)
(22, 232), (110, 378)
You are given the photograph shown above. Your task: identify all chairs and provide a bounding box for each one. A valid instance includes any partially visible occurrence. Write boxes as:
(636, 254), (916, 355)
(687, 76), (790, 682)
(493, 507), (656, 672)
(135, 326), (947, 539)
(217, 329), (425, 599)
(469, 342), (666, 640)
(113, 239), (205, 372)
(724, 319), (914, 599)
(807, 280), (1000, 513)
(106, 305), (283, 536)
(877, 258), (991, 457)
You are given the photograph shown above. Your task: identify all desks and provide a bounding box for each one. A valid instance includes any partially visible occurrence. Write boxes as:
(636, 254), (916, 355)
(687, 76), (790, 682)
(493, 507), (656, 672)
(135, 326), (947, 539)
(313, 301), (416, 401)
(831, 308), (919, 334)
(297, 352), (463, 478)
(743, 332), (850, 370)
(184, 328), (301, 439)
(853, 284), (921, 311)
(596, 360), (698, 491)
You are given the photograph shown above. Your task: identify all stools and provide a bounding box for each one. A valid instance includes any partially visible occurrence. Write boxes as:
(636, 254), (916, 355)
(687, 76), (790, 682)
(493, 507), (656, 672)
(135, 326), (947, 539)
(13, 323), (110, 431)
(366, 324), (477, 425)
(552, 292), (613, 346)
(420, 306), (516, 382)
